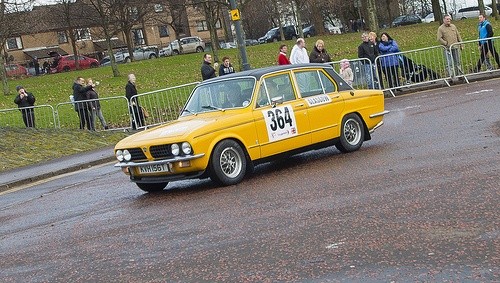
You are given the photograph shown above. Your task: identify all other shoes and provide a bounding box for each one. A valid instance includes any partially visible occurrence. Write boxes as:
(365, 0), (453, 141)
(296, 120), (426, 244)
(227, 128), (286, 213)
(104, 126), (109, 130)
(139, 126), (149, 129)
(133, 128), (140, 131)
(458, 77), (465, 80)
(397, 87), (403, 92)
(390, 90), (395, 92)
(469, 68), (480, 72)
(448, 76), (453, 82)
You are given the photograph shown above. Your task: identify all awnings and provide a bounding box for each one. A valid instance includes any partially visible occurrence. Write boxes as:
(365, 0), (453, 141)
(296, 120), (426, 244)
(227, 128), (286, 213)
(92, 40), (127, 50)
(23, 45), (69, 59)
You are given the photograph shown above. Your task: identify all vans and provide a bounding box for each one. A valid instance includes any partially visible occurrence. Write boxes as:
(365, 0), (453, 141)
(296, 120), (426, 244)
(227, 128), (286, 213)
(258, 25), (297, 44)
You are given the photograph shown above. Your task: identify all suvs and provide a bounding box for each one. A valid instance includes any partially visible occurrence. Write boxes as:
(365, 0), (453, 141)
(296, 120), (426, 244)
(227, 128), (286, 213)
(455, 6), (500, 21)
(159, 35), (206, 58)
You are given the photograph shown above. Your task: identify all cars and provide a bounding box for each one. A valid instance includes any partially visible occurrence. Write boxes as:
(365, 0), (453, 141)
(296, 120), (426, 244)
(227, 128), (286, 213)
(297, 25), (341, 40)
(99, 50), (158, 67)
(0, 63), (43, 79)
(113, 63), (390, 193)
(219, 39), (258, 50)
(46, 51), (100, 75)
(381, 13), (435, 28)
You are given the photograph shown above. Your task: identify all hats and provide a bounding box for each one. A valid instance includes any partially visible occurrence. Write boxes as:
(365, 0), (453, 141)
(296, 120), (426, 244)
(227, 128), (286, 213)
(16, 85), (24, 92)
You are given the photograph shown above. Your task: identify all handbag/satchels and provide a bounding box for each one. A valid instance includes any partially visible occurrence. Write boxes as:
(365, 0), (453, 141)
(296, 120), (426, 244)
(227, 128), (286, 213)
(69, 95), (75, 106)
(141, 106), (149, 118)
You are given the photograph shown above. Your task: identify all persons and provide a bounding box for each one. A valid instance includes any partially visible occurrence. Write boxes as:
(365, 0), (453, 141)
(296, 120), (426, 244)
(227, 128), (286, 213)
(290, 38), (309, 64)
(470, 14), (500, 73)
(2, 52), (8, 59)
(72, 77), (95, 130)
(278, 44), (291, 65)
(201, 53), (217, 105)
(437, 14), (463, 77)
(85, 78), (108, 131)
(33, 56), (40, 76)
(358, 32), (377, 89)
(219, 57), (235, 89)
(14, 85), (35, 127)
(369, 32), (386, 92)
(339, 59), (354, 85)
(379, 33), (403, 92)
(8, 55), (14, 62)
(125, 74), (149, 130)
(310, 39), (333, 88)
